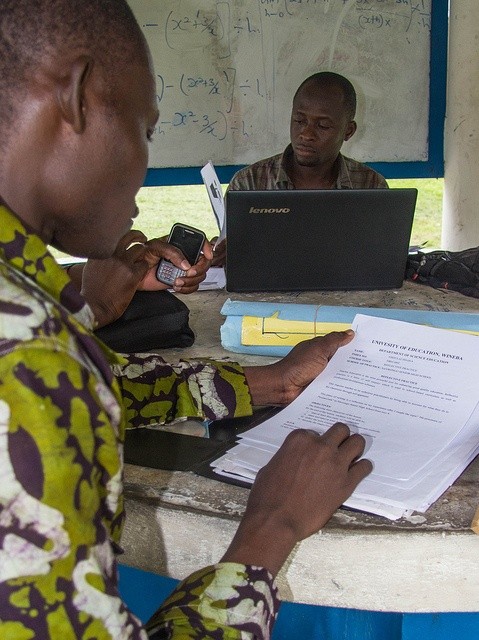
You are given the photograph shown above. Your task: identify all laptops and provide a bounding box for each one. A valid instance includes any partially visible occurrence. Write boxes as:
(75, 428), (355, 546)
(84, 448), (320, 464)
(227, 188), (418, 292)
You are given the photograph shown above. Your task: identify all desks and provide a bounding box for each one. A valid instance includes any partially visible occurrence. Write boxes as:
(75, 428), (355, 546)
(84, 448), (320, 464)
(113, 278), (479, 614)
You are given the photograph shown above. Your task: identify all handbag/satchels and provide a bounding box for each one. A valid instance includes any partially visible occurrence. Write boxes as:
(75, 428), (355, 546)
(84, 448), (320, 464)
(94, 290), (195, 353)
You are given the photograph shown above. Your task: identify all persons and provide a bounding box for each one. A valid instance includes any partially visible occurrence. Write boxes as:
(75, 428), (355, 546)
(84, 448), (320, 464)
(53, 229), (214, 329)
(0, 1), (370, 640)
(207, 71), (391, 266)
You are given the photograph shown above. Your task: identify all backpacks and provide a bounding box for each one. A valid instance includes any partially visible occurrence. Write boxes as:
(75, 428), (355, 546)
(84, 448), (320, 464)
(405, 246), (479, 299)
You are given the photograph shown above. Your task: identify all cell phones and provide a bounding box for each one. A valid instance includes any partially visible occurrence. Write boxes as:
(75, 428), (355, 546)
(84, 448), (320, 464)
(156, 223), (206, 287)
